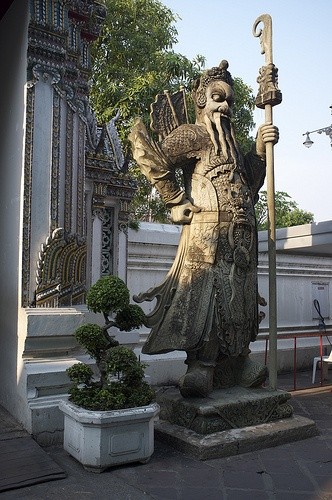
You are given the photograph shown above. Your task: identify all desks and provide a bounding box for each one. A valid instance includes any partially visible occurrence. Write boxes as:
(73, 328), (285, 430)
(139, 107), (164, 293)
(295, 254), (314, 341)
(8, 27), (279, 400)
(257, 329), (332, 392)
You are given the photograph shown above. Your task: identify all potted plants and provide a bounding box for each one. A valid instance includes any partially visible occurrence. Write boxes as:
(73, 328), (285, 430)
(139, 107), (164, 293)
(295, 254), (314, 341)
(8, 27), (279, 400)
(57, 273), (161, 475)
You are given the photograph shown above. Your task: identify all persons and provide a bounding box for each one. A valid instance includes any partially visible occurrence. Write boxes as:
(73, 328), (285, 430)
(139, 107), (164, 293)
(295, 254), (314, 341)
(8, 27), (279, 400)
(127, 60), (279, 401)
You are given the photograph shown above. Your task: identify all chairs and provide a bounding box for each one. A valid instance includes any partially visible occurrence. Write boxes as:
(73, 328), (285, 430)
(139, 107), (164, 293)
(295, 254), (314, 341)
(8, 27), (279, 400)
(311, 350), (332, 385)
